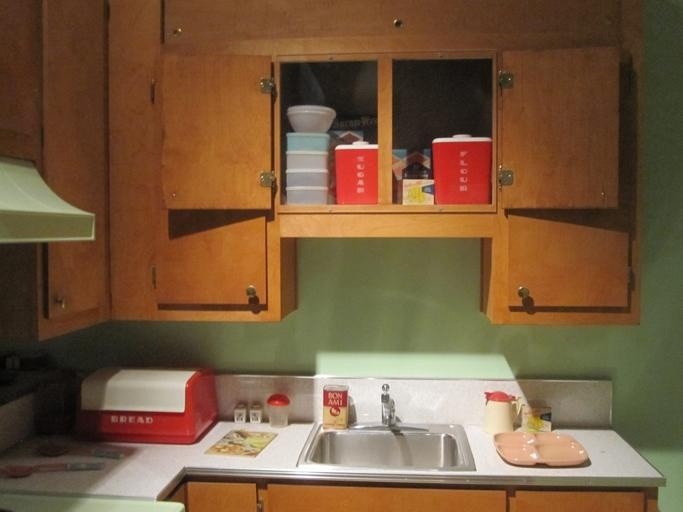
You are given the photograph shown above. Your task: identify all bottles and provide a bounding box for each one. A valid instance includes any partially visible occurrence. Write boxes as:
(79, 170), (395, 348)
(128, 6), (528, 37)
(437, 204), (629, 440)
(232, 401), (248, 424)
(247, 400), (265, 427)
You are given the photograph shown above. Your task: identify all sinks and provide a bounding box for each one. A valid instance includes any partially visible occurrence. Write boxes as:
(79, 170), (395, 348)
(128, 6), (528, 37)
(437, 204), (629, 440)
(295, 420), (477, 475)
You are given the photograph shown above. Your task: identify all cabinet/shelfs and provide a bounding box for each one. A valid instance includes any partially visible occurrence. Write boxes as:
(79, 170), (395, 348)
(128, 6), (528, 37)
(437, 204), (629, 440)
(482, 43), (643, 328)
(0, 2), (109, 343)
(170, 479), (658, 512)
(161, 0), (640, 56)
(106, 1), (299, 325)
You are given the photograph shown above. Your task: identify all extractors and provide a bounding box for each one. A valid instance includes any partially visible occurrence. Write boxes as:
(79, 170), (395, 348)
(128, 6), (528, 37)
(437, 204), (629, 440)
(0, 156), (95, 247)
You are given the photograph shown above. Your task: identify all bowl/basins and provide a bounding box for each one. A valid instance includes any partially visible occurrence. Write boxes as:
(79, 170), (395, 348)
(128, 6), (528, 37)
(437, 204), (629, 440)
(284, 104), (338, 136)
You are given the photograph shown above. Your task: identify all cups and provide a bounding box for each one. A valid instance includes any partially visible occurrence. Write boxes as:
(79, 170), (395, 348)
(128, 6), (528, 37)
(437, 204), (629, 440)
(265, 391), (291, 429)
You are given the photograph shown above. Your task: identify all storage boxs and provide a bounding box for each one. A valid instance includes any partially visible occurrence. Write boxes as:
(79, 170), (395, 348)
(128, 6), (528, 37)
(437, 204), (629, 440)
(66, 366), (221, 444)
(430, 131), (492, 206)
(331, 139), (378, 209)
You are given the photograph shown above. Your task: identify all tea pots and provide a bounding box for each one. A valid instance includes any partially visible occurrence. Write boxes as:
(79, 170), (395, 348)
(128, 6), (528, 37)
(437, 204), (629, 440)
(482, 390), (522, 437)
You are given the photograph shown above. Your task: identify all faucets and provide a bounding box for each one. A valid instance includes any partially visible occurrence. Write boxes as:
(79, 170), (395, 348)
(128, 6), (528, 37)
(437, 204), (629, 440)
(379, 383), (401, 428)
